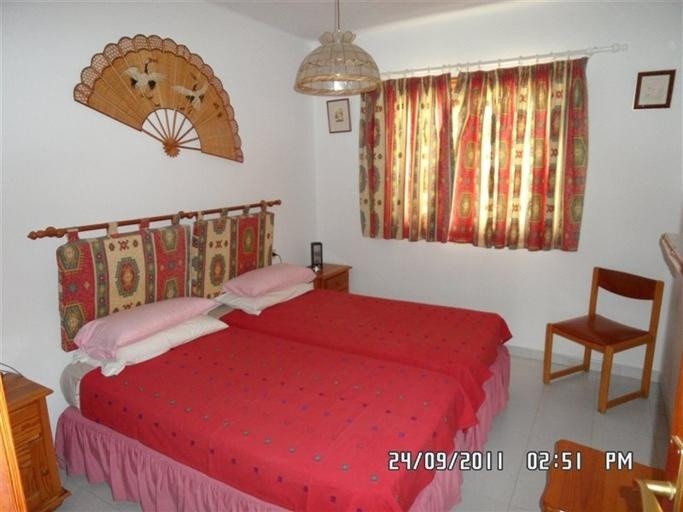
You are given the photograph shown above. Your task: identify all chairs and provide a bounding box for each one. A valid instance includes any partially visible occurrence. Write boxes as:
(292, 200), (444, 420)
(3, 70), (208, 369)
(543, 266), (664, 413)
(539, 439), (668, 512)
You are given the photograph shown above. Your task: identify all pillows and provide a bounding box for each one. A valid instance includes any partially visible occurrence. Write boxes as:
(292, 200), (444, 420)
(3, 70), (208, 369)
(73, 315), (230, 377)
(215, 282), (315, 316)
(73, 296), (223, 363)
(221, 263), (317, 299)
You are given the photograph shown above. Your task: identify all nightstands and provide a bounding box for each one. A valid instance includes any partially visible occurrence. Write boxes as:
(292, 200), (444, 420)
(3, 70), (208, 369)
(28, 200), (513, 512)
(307, 260), (352, 294)
(0, 366), (72, 512)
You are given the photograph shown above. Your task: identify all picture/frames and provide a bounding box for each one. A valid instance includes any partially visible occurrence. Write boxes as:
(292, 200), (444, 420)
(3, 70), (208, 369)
(632, 69), (677, 109)
(326, 98), (351, 134)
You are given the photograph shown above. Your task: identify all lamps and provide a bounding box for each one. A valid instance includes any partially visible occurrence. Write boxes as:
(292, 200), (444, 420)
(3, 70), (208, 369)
(293, 0), (381, 97)
